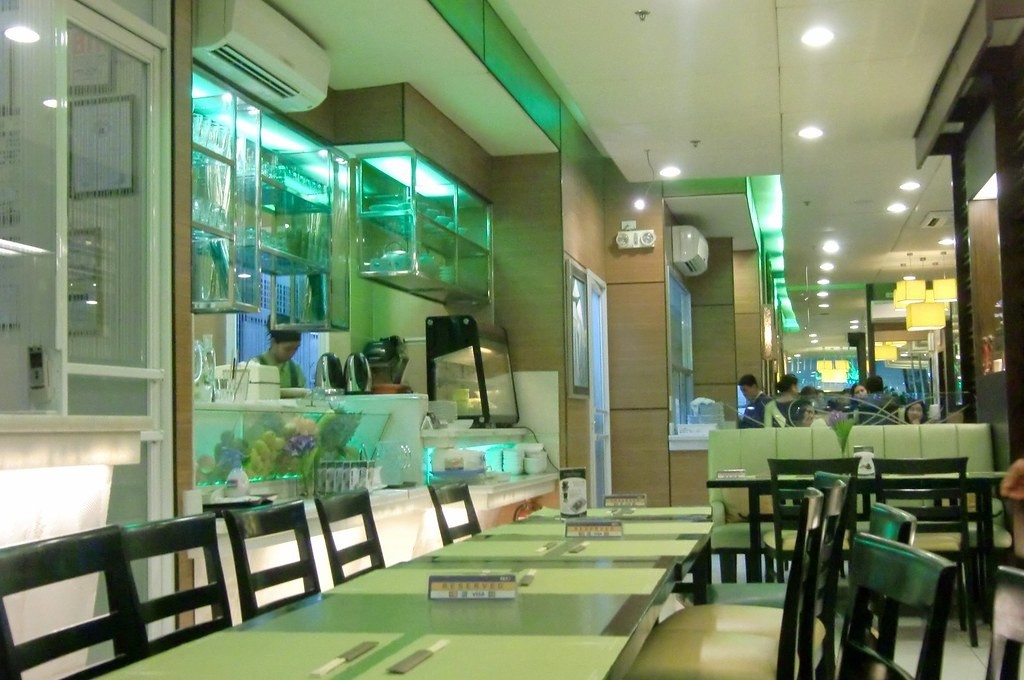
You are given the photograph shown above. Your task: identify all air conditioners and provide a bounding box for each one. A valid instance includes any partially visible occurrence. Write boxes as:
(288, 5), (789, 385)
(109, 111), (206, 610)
(189, 0), (330, 114)
(672, 225), (708, 276)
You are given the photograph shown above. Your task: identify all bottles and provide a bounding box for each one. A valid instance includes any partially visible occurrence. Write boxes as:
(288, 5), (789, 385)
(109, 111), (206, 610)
(261, 159), (328, 193)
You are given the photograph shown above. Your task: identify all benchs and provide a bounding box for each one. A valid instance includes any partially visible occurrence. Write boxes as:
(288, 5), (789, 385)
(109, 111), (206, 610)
(708, 422), (1013, 615)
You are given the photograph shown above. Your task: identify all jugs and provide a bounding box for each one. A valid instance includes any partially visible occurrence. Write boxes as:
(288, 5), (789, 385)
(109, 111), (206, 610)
(418, 242), (436, 277)
(379, 241), (410, 271)
(193, 340), (217, 404)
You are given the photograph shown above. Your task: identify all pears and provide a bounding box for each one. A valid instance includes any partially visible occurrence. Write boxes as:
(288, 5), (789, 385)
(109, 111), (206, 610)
(243, 421), (299, 479)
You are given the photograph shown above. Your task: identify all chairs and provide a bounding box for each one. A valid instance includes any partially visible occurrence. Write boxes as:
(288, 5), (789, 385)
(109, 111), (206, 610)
(428, 479), (481, 546)
(623, 457), (1024, 680)
(222, 499), (320, 623)
(0, 515), (233, 679)
(317, 488), (384, 586)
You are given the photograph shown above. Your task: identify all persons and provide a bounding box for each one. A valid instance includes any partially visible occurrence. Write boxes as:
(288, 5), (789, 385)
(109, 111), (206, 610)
(253, 314), (311, 398)
(738, 373), (927, 429)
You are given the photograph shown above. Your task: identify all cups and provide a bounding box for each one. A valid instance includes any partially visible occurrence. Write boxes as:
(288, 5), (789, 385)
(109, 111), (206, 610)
(192, 114), (232, 158)
(192, 195), (226, 229)
(215, 368), (250, 402)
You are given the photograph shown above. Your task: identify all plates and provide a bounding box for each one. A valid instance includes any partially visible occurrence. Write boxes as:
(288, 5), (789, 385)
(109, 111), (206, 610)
(367, 201), (397, 211)
(439, 266), (487, 295)
(429, 400), (457, 429)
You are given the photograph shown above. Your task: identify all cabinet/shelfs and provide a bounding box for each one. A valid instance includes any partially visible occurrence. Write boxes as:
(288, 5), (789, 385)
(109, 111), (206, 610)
(191, 62), (351, 329)
(355, 149), (491, 305)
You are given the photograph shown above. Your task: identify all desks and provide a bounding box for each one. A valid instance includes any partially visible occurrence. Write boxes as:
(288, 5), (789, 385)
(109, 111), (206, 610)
(91, 507), (713, 680)
(707, 469), (1008, 624)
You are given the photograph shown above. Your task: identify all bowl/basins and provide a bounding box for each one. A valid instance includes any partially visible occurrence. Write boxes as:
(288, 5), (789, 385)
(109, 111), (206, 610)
(398, 203), (467, 235)
(485, 442), (547, 475)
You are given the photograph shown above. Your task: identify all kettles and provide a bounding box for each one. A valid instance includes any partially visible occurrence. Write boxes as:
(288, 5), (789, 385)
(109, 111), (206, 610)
(344, 352), (373, 395)
(315, 353), (345, 395)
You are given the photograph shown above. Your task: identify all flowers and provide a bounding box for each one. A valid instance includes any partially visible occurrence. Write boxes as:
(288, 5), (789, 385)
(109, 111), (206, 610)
(828, 411), (853, 453)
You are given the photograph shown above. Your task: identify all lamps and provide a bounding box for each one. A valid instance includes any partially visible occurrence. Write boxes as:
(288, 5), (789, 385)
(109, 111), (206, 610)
(817, 346), (850, 382)
(616, 229), (657, 248)
(892, 271), (958, 332)
(874, 341), (907, 361)
